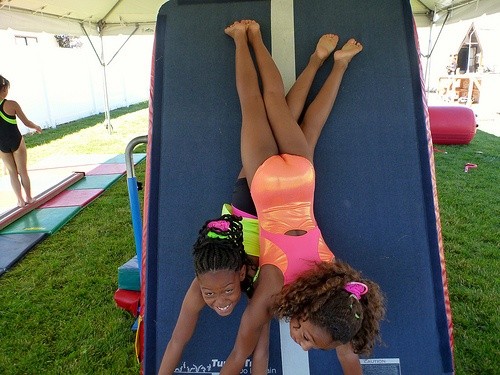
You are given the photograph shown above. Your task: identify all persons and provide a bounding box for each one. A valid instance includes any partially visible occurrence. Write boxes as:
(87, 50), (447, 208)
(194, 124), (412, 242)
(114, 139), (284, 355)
(220, 20), (386, 375)
(156, 34), (363, 375)
(0, 75), (42, 208)
